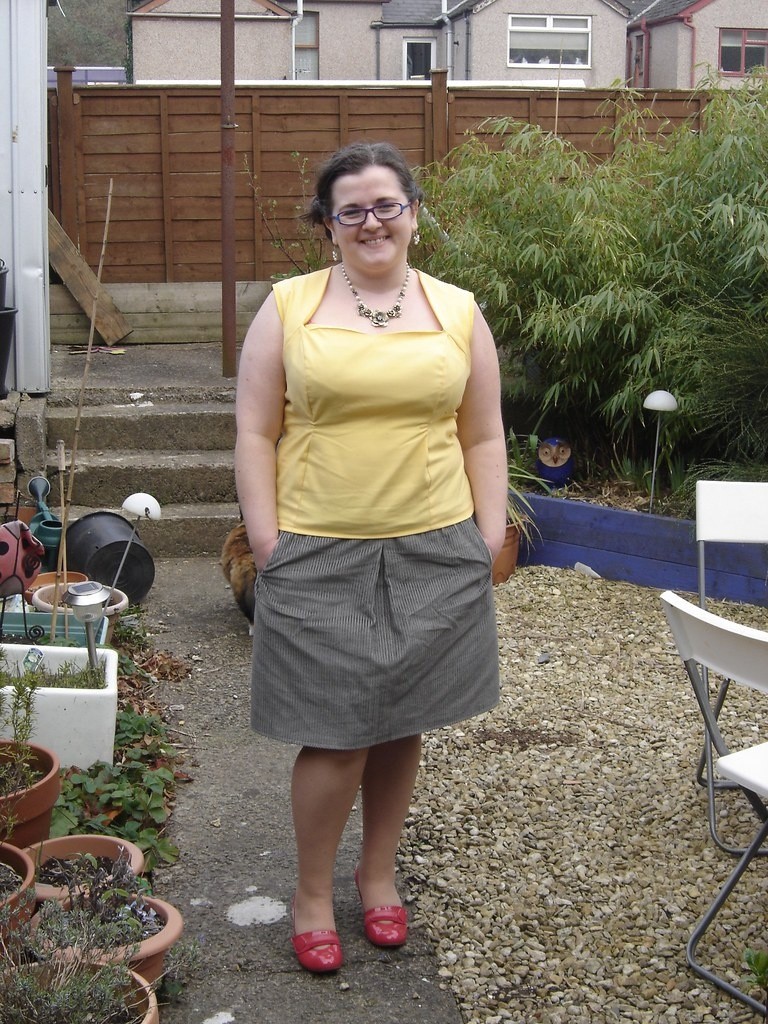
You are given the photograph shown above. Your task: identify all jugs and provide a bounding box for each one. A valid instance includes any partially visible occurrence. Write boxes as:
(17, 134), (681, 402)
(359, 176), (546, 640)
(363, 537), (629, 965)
(27, 476), (64, 575)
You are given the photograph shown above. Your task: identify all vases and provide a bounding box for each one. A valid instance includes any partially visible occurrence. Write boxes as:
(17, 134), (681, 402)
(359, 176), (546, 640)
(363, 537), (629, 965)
(24, 510), (154, 645)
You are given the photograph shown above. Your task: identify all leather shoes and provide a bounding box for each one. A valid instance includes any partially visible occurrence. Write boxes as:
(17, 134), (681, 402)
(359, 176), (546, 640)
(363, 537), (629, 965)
(293, 891), (342, 972)
(355, 861), (408, 947)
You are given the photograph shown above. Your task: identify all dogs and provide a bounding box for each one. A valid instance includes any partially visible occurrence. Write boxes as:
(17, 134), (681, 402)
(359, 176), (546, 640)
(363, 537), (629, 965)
(220, 506), (259, 635)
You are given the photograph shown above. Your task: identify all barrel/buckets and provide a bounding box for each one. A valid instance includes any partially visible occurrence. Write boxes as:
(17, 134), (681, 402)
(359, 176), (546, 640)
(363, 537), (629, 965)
(65, 510), (155, 605)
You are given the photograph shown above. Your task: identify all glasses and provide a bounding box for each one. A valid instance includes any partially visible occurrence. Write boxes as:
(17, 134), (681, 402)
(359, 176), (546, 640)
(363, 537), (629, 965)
(326, 198), (414, 226)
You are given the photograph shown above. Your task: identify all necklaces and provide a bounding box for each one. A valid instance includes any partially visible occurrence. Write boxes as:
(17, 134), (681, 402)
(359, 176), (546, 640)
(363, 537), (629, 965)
(341, 261), (410, 328)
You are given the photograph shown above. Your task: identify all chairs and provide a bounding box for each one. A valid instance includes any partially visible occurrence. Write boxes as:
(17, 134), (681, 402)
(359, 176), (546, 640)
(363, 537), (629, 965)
(659, 480), (768, 1016)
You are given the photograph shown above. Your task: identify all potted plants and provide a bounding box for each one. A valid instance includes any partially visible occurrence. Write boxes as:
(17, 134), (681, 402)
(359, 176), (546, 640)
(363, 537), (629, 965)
(491, 434), (553, 583)
(0, 613), (184, 1024)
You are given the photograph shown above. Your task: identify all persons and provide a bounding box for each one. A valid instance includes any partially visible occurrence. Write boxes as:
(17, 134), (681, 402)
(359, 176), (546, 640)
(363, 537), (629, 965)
(236, 142), (509, 968)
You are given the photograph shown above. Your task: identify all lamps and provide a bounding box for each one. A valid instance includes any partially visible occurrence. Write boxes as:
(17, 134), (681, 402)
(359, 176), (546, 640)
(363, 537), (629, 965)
(62, 582), (111, 624)
